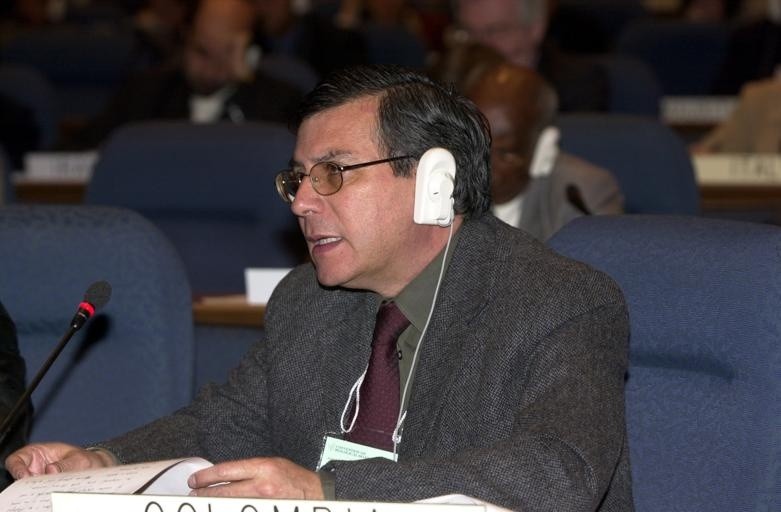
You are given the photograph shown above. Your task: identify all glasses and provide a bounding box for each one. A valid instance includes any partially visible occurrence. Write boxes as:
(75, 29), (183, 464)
(275, 154), (410, 204)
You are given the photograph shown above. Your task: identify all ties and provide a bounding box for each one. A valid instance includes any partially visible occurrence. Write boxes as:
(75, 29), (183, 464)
(343, 302), (412, 452)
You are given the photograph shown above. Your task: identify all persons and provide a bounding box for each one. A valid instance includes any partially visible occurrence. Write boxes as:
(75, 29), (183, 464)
(3, 66), (639, 511)
(1, 0), (780, 248)
(0, 302), (37, 489)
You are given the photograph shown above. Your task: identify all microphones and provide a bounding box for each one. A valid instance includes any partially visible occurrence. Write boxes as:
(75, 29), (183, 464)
(565, 183), (592, 215)
(2, 280), (113, 437)
